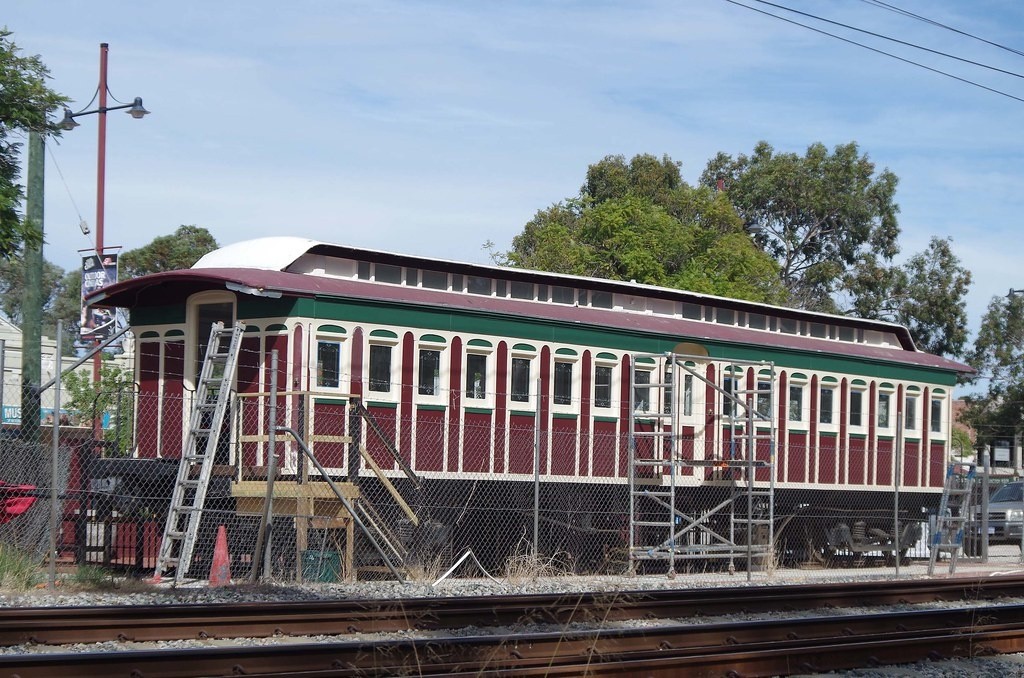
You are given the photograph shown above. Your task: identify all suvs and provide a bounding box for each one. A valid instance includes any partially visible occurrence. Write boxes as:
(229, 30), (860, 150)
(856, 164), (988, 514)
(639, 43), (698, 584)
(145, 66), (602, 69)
(964, 480), (1023, 557)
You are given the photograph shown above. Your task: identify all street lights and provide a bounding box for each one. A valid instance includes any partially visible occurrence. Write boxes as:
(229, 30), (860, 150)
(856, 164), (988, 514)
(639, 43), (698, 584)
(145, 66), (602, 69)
(56, 41), (148, 452)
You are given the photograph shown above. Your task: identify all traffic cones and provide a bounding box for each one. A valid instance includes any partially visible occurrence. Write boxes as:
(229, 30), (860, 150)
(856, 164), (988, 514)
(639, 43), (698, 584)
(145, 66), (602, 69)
(206, 527), (242, 591)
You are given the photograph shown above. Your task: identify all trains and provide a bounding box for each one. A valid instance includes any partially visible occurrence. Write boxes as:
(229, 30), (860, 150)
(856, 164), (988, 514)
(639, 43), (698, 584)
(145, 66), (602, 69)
(81, 235), (970, 574)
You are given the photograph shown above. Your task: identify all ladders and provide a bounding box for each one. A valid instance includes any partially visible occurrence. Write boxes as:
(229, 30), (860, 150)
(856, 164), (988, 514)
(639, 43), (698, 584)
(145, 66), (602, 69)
(927, 460), (977, 578)
(151, 318), (248, 586)
